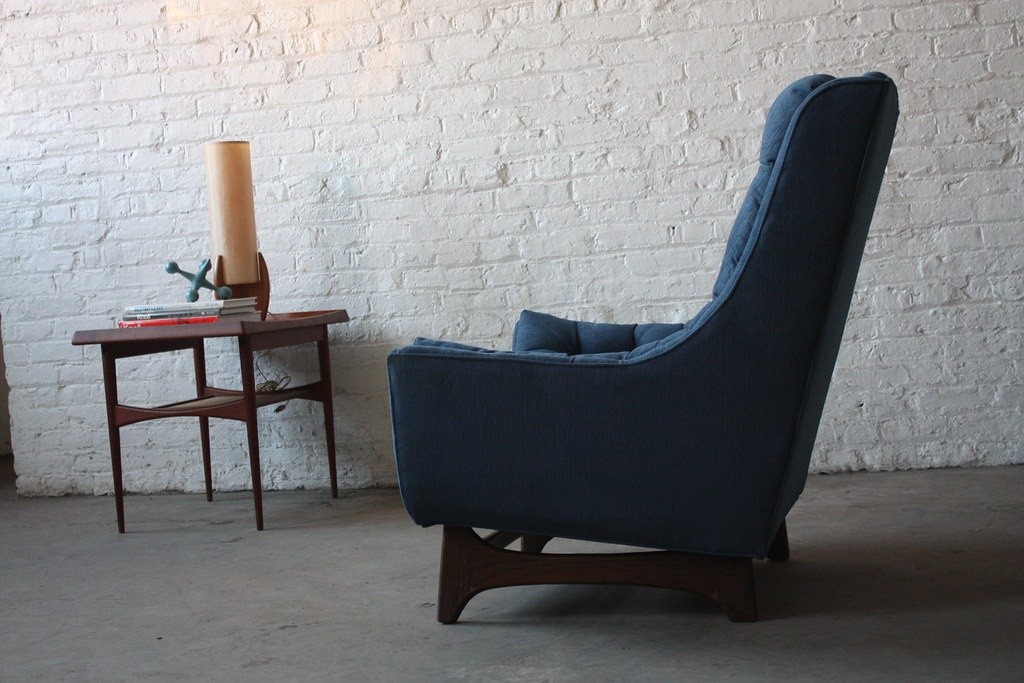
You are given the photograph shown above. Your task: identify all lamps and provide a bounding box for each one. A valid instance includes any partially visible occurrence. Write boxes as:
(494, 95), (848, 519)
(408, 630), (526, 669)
(203, 140), (270, 319)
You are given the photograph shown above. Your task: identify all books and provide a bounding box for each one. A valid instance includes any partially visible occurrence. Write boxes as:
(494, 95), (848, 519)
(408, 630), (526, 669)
(118, 297), (262, 328)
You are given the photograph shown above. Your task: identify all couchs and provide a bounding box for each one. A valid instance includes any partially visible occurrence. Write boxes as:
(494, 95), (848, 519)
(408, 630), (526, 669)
(387, 72), (900, 623)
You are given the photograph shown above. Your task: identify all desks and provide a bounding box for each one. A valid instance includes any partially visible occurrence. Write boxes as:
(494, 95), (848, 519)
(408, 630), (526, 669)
(71, 310), (349, 534)
